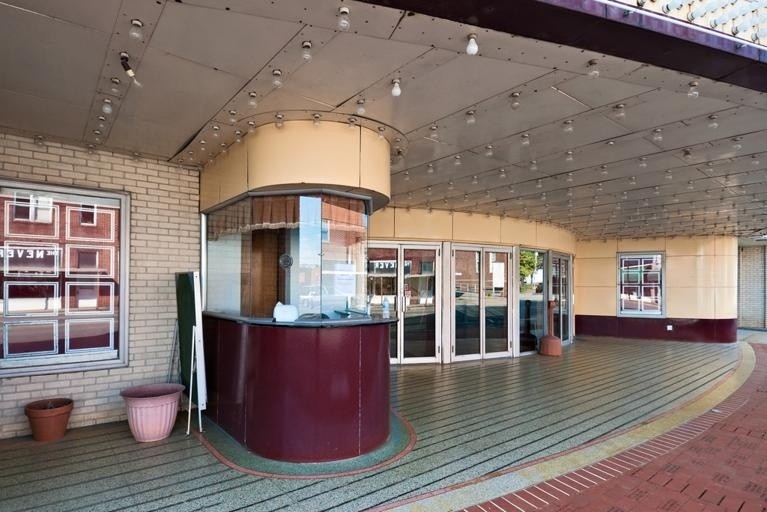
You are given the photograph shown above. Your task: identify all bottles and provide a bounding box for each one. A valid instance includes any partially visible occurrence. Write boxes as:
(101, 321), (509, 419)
(382, 297), (389, 319)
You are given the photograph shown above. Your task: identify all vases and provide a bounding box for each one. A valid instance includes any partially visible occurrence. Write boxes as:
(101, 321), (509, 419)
(24, 398), (73, 442)
(119, 383), (184, 443)
